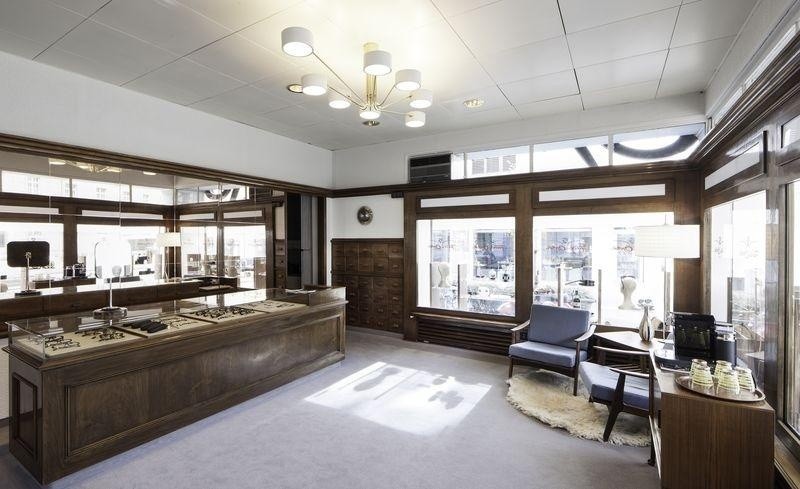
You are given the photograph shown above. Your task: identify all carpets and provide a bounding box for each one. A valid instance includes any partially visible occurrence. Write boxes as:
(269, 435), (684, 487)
(505, 369), (662, 447)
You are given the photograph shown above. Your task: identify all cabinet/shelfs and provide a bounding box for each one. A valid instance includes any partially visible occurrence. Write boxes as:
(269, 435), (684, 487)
(331, 238), (406, 334)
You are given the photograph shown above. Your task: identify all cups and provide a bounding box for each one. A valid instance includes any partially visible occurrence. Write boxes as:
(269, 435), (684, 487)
(736, 367), (757, 392)
(714, 360), (732, 379)
(690, 359), (707, 379)
(719, 370), (740, 392)
(690, 365), (714, 386)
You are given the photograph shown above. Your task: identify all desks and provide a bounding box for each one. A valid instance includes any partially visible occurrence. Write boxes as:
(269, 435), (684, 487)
(655, 373), (776, 489)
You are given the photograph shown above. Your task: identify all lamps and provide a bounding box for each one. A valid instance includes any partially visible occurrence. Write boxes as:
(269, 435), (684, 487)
(275, 26), (433, 128)
(633, 212), (700, 342)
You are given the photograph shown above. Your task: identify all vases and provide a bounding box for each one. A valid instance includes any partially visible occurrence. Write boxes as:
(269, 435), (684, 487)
(640, 310), (656, 344)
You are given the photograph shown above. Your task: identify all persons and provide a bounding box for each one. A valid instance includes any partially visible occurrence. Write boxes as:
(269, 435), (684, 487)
(438, 262), (451, 288)
(619, 277), (641, 310)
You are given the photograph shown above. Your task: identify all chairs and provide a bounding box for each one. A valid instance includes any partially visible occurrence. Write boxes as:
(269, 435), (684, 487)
(508, 304), (597, 396)
(577, 345), (664, 442)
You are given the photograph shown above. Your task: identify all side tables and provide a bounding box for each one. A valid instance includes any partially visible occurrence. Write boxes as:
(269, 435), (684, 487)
(594, 331), (666, 372)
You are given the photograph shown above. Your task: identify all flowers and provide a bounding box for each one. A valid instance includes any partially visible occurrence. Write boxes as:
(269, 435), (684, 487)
(639, 297), (656, 311)
